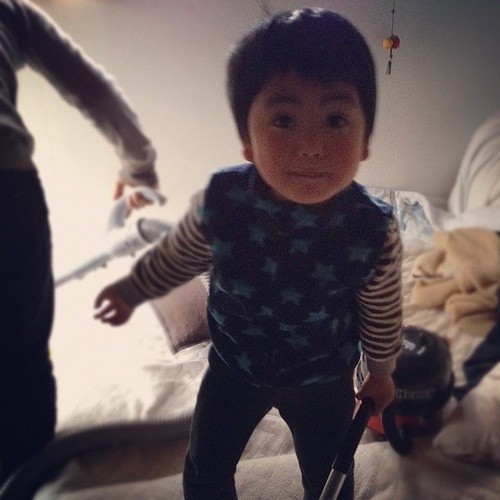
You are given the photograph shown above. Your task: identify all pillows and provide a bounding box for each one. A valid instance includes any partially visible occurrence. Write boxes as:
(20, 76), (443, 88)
(148, 270), (210, 353)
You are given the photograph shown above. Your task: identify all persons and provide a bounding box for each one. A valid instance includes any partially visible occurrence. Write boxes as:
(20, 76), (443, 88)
(1, 1), (164, 500)
(92, 8), (403, 497)
